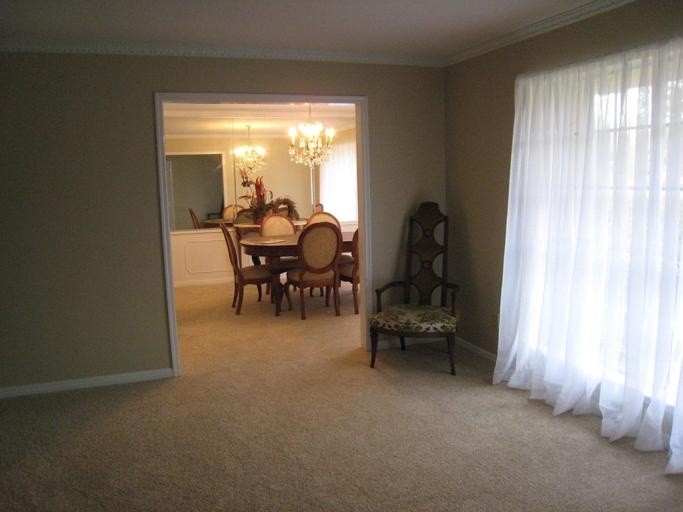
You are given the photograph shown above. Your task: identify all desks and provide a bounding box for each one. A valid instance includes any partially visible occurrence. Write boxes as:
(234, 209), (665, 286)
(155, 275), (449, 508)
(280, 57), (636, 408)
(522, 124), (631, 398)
(202, 218), (224, 227)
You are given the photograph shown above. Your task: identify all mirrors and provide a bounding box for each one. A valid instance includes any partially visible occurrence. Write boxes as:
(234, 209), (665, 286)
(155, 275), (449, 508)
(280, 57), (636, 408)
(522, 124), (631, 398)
(164, 151), (225, 230)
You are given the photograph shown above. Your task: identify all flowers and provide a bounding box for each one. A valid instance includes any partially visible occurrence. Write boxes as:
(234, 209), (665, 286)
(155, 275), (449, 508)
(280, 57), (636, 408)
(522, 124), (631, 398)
(227, 169), (299, 220)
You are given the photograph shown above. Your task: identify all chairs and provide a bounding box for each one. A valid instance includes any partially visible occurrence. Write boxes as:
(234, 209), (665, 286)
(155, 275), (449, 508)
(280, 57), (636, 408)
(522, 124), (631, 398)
(218, 213), (359, 320)
(189, 208), (201, 230)
(369, 201), (458, 375)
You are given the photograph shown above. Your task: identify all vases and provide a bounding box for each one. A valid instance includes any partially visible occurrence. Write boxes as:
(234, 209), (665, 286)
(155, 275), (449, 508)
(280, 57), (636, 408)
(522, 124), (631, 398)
(254, 210), (265, 225)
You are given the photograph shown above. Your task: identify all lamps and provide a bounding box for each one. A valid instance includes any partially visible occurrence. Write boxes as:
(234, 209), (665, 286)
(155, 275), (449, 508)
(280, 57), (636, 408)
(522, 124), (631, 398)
(287, 105), (336, 167)
(230, 126), (266, 174)
